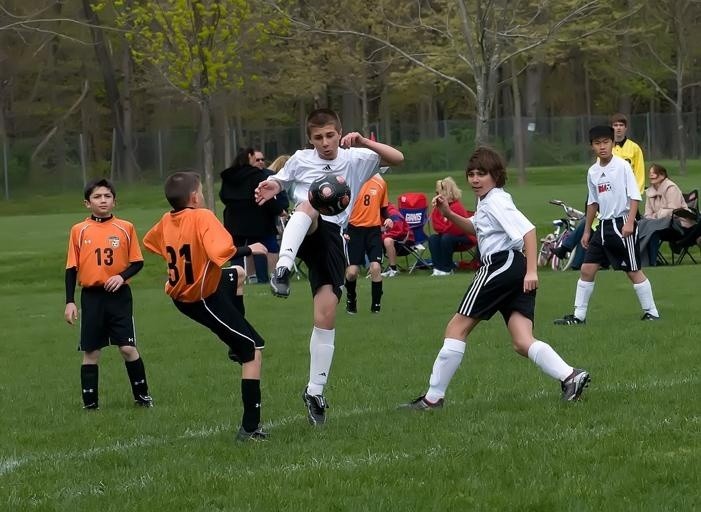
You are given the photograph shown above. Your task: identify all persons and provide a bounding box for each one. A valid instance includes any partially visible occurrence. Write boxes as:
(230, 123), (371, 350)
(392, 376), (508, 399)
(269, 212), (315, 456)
(217, 149), (267, 284)
(596, 113), (645, 196)
(343, 172), (393, 315)
(255, 108), (404, 425)
(380, 202), (415, 278)
(252, 148), (289, 252)
(394, 146), (591, 412)
(246, 148), (290, 284)
(66, 178), (154, 410)
(553, 125), (660, 325)
(551, 192), (599, 270)
(143, 171), (270, 444)
(645, 163), (689, 266)
(267, 155), (291, 173)
(267, 155), (289, 209)
(429, 177), (477, 276)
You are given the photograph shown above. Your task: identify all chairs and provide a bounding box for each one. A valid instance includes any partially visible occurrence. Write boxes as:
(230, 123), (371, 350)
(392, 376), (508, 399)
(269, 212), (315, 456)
(656, 192), (699, 265)
(452, 210), (479, 268)
(383, 192), (432, 275)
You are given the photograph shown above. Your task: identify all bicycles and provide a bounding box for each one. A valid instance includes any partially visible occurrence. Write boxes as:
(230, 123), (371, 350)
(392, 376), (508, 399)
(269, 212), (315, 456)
(536, 199), (586, 272)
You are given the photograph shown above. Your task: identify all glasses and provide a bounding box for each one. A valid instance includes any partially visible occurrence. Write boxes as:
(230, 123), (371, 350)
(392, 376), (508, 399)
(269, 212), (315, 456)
(255, 158), (265, 161)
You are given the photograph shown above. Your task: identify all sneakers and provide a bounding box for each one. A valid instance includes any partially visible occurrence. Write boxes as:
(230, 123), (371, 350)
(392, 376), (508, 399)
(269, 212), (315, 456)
(345, 300), (357, 315)
(381, 267), (400, 278)
(249, 276), (257, 284)
(236, 427), (268, 444)
(302, 386), (329, 426)
(438, 271), (452, 276)
(640, 313), (661, 320)
(549, 247), (566, 260)
(134, 395), (154, 408)
(398, 395), (444, 410)
(555, 314), (586, 326)
(560, 368), (591, 402)
(431, 269), (440, 276)
(370, 304), (382, 314)
(270, 266), (291, 299)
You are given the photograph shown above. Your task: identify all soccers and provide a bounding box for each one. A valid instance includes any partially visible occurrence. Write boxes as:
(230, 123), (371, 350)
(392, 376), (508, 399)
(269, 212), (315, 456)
(308, 173), (352, 216)
